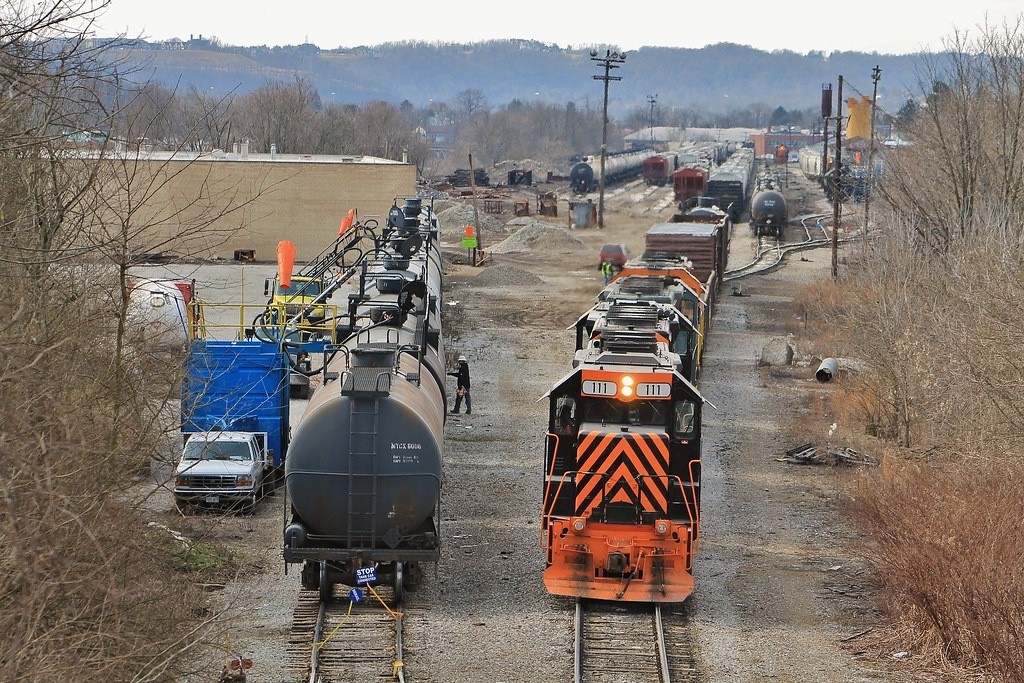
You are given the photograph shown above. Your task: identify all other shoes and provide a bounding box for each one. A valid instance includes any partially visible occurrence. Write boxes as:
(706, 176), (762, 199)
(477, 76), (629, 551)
(450, 410), (459, 414)
(466, 410), (471, 414)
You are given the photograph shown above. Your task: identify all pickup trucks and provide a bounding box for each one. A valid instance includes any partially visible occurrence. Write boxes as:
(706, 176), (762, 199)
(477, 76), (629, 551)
(242, 321), (311, 398)
(173, 338), (291, 517)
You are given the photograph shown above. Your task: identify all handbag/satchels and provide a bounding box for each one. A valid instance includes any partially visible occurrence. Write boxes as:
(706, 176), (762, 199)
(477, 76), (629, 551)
(456, 385), (467, 397)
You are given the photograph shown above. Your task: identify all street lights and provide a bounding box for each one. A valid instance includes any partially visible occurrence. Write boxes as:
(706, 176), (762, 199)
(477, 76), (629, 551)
(589, 47), (626, 227)
(861, 61), (882, 262)
(646, 93), (660, 139)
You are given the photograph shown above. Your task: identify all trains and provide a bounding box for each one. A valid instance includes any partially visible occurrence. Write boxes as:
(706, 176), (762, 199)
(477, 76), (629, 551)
(570, 139), (887, 240)
(282, 196), (448, 603)
(535, 206), (733, 606)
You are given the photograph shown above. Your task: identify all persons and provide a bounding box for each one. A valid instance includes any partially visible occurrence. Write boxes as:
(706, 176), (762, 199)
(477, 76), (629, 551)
(446, 355), (474, 415)
(601, 258), (613, 285)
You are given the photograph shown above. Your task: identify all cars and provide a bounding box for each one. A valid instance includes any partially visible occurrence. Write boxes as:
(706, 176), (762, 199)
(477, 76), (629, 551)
(761, 122), (845, 136)
(595, 242), (630, 270)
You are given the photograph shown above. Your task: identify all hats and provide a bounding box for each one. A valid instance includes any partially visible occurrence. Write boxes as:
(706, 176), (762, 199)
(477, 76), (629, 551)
(458, 356), (466, 361)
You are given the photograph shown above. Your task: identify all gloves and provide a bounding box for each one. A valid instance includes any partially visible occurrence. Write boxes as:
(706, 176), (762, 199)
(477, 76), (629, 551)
(458, 387), (463, 390)
(447, 373), (451, 375)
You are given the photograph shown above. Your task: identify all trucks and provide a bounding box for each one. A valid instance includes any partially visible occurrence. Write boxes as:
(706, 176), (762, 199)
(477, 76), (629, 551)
(264, 269), (329, 329)
(122, 277), (199, 374)
